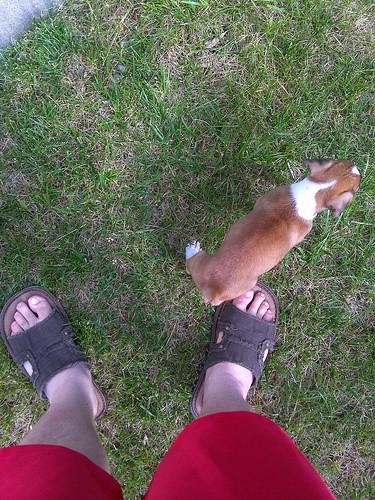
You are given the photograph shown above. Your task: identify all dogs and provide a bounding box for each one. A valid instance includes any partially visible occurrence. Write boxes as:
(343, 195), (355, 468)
(185, 157), (361, 306)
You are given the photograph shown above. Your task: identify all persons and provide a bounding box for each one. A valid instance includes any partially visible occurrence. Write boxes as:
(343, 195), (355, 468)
(1, 278), (337, 500)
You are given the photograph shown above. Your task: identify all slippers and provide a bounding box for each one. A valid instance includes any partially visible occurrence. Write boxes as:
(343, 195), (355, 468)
(0, 286), (108, 421)
(190, 281), (279, 420)
